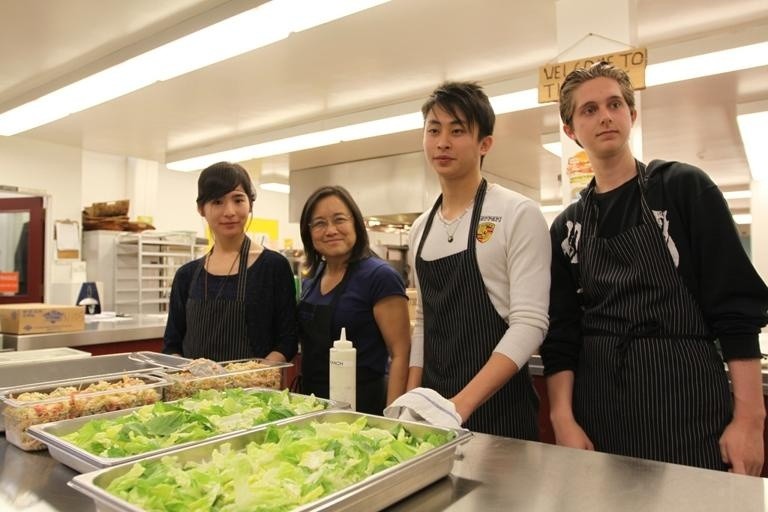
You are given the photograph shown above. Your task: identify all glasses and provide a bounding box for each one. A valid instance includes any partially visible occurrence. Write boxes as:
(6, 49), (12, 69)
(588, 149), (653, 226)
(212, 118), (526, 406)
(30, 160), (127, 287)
(307, 215), (351, 233)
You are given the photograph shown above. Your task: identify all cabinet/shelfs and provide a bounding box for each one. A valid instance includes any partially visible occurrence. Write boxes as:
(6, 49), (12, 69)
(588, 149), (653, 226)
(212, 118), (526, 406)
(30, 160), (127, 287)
(114, 237), (195, 318)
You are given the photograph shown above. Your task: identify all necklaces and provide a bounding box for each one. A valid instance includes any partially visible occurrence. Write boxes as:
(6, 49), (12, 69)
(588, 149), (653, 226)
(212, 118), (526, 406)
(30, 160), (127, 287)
(444, 199), (474, 243)
(203, 247), (240, 301)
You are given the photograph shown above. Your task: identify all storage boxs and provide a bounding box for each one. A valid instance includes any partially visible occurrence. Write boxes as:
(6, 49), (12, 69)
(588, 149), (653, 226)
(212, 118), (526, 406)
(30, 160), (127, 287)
(151, 357), (295, 402)
(0, 373), (175, 452)
(0, 303), (87, 334)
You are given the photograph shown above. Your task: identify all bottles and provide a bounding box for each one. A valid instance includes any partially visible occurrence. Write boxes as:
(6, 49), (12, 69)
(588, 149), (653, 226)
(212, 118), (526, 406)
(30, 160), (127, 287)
(403, 286), (417, 320)
(326, 324), (357, 410)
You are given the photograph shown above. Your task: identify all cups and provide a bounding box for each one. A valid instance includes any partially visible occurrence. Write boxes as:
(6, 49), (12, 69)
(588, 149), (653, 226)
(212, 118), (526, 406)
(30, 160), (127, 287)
(246, 231), (267, 245)
(283, 233), (293, 249)
(137, 216), (153, 225)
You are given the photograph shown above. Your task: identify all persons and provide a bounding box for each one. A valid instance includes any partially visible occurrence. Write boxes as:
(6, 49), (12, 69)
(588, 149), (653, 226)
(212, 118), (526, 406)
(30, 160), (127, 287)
(539, 61), (768, 478)
(161, 162), (299, 363)
(292, 185), (412, 418)
(404, 79), (552, 443)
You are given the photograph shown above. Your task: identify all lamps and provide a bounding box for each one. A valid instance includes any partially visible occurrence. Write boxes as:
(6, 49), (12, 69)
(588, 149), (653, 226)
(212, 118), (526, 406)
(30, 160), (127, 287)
(734, 101), (767, 181)
(258, 175), (291, 193)
(540, 133), (562, 158)
